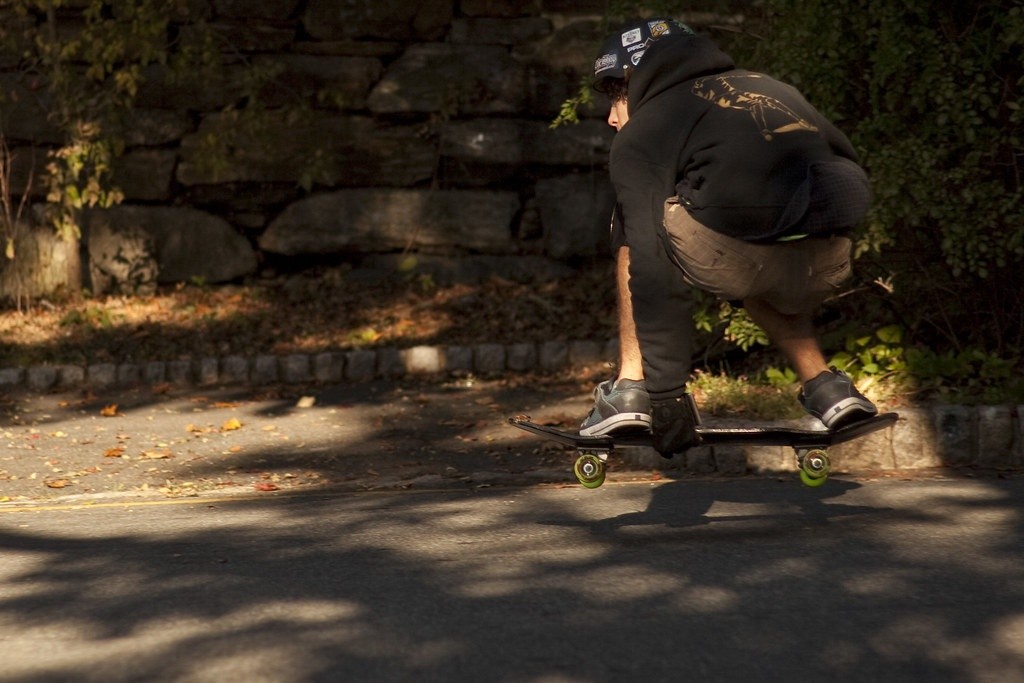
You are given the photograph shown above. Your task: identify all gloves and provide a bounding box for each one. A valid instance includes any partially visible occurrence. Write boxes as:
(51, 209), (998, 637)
(652, 393), (700, 460)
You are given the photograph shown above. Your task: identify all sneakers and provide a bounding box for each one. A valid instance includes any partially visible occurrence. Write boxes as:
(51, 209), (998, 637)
(579, 376), (653, 442)
(797, 366), (878, 430)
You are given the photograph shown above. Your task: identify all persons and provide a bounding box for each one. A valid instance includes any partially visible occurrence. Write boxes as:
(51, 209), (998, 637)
(575, 16), (880, 458)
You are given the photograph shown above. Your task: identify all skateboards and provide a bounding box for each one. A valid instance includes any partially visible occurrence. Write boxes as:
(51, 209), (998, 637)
(506, 412), (900, 490)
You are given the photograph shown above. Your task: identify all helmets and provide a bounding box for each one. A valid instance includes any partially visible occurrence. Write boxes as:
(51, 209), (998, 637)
(595, 17), (696, 78)
